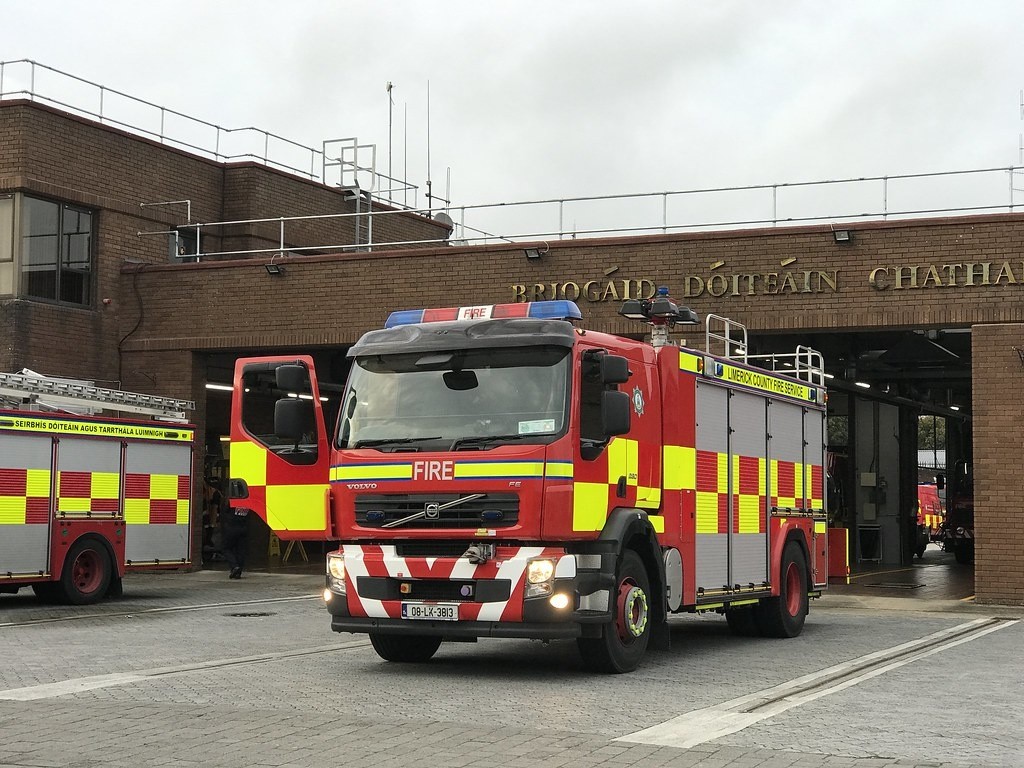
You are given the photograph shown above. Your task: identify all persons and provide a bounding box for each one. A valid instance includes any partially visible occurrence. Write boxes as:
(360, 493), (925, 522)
(219, 496), (249, 579)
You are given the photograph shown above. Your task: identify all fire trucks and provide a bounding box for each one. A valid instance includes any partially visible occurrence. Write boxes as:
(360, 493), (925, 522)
(0, 369), (198, 608)
(228, 299), (850, 675)
(916, 484), (946, 559)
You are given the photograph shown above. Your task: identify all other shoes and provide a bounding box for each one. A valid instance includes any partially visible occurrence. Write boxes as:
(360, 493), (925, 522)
(230, 566), (242, 579)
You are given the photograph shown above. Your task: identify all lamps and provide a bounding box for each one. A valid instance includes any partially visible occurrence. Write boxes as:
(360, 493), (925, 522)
(265, 263), (280, 274)
(206, 382), (250, 393)
(833, 229), (852, 243)
(283, 391), (329, 402)
(524, 247), (540, 259)
